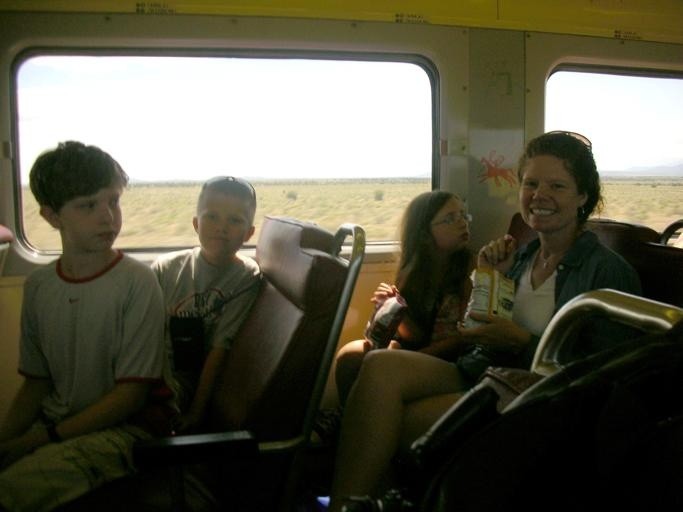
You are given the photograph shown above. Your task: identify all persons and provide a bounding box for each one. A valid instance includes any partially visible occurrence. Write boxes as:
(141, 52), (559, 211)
(150, 176), (263, 413)
(0, 141), (179, 510)
(324, 131), (643, 511)
(334, 191), (480, 405)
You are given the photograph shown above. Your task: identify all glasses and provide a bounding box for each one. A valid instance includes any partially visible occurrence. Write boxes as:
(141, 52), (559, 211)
(431, 212), (471, 226)
(550, 130), (592, 152)
(205, 176), (254, 198)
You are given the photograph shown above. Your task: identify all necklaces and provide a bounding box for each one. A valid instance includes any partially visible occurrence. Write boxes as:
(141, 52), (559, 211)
(537, 252), (560, 271)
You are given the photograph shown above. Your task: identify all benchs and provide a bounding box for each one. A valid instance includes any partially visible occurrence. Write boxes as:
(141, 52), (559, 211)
(477, 288), (681, 426)
(504, 199), (681, 284)
(153, 211), (366, 507)
